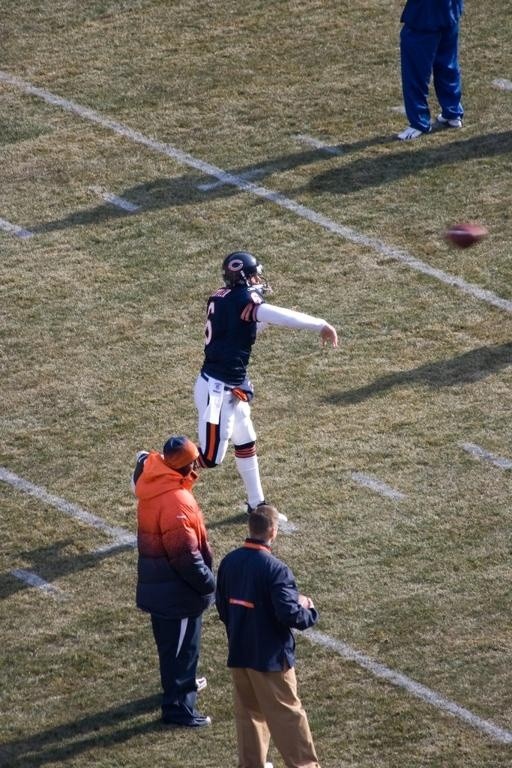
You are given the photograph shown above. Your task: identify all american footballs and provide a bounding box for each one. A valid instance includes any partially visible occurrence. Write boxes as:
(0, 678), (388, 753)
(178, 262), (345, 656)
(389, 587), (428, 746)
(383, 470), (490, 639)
(446, 224), (487, 250)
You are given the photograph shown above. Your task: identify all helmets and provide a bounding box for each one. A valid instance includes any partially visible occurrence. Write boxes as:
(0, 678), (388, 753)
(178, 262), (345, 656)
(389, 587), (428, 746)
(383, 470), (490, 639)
(221, 251), (264, 289)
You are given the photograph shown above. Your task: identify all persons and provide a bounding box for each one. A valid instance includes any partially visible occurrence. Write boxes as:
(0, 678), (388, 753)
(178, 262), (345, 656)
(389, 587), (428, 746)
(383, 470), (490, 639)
(214, 503), (322, 768)
(132, 436), (217, 728)
(391, 0), (464, 141)
(132, 250), (339, 521)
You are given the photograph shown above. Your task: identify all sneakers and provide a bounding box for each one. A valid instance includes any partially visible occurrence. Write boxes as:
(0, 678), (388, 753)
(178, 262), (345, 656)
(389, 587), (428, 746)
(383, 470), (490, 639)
(245, 500), (288, 526)
(159, 712), (212, 729)
(437, 113), (462, 128)
(195, 677), (208, 692)
(131, 450), (150, 496)
(397, 125), (433, 140)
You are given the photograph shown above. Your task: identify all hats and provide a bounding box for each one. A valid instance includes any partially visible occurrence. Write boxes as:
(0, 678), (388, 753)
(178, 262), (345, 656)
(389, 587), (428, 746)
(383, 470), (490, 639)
(162, 436), (200, 470)
